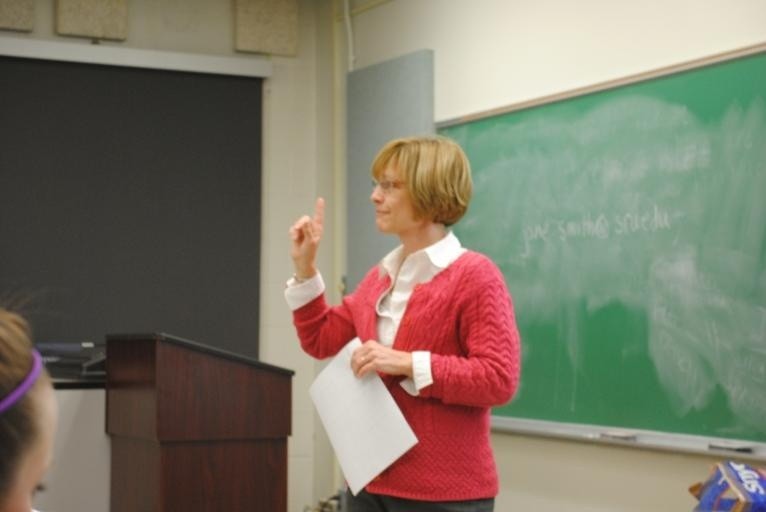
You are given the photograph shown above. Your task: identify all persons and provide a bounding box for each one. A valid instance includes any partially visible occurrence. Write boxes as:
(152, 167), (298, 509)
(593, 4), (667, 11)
(0, 307), (60, 512)
(283, 134), (522, 512)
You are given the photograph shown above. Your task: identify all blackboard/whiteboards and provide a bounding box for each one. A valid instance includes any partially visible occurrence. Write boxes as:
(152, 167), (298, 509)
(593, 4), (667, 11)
(1, 38), (264, 390)
(437, 45), (764, 464)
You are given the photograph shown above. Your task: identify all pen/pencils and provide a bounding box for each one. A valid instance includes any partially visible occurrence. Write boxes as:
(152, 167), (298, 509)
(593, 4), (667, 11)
(601, 433), (637, 440)
(707, 443), (754, 454)
